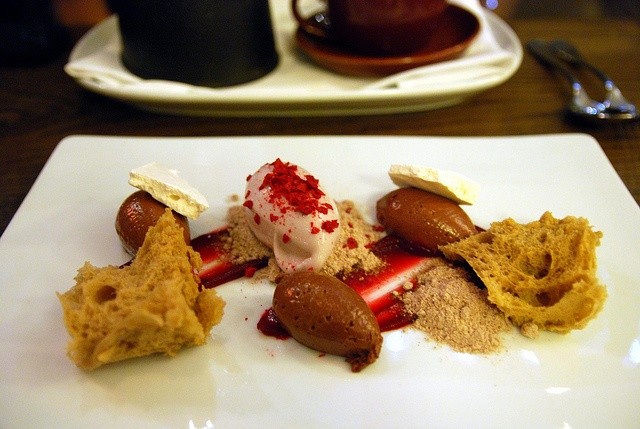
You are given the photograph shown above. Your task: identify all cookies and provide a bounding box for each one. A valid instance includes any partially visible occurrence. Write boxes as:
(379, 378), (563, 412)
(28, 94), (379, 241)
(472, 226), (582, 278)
(128, 164), (210, 221)
(388, 163), (475, 207)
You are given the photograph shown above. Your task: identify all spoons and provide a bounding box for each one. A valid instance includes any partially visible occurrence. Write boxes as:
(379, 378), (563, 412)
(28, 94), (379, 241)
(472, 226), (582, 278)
(523, 38), (605, 115)
(546, 38), (637, 119)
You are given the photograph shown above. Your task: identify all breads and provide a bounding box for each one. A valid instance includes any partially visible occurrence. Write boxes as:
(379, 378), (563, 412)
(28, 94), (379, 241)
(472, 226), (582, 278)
(438, 210), (607, 337)
(57, 209), (226, 370)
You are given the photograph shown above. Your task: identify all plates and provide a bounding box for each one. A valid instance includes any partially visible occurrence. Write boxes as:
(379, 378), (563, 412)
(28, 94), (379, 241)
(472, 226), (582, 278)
(297, 2), (481, 75)
(0, 134), (640, 429)
(61, 1), (524, 117)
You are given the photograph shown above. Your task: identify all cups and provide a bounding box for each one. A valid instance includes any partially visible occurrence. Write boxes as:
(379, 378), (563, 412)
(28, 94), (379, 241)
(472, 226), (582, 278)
(292, 0), (446, 55)
